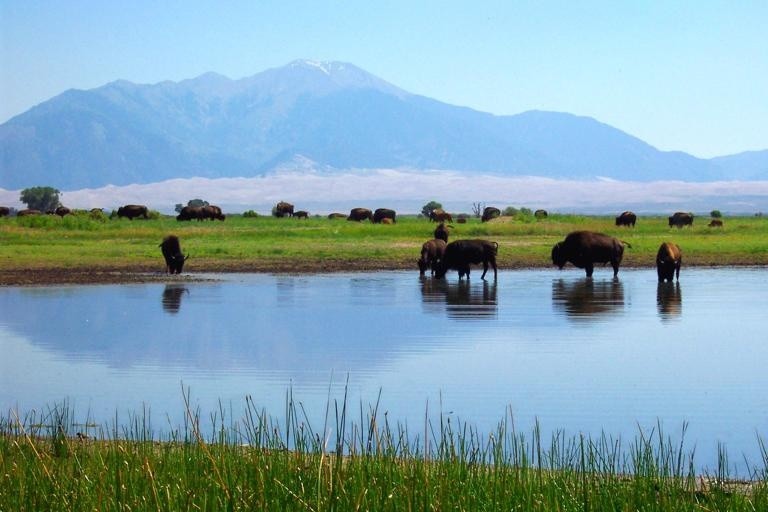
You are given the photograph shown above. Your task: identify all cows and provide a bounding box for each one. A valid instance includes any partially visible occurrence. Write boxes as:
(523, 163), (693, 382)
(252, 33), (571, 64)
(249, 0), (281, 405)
(615, 210), (636, 228)
(707, 220), (722, 227)
(156, 233), (189, 276)
(416, 206), (502, 283)
(275, 200), (308, 219)
(176, 205), (225, 223)
(551, 231), (633, 279)
(1, 205), (70, 218)
(533, 208), (549, 218)
(655, 241), (684, 280)
(328, 207), (396, 225)
(117, 204), (148, 220)
(668, 212), (694, 229)
